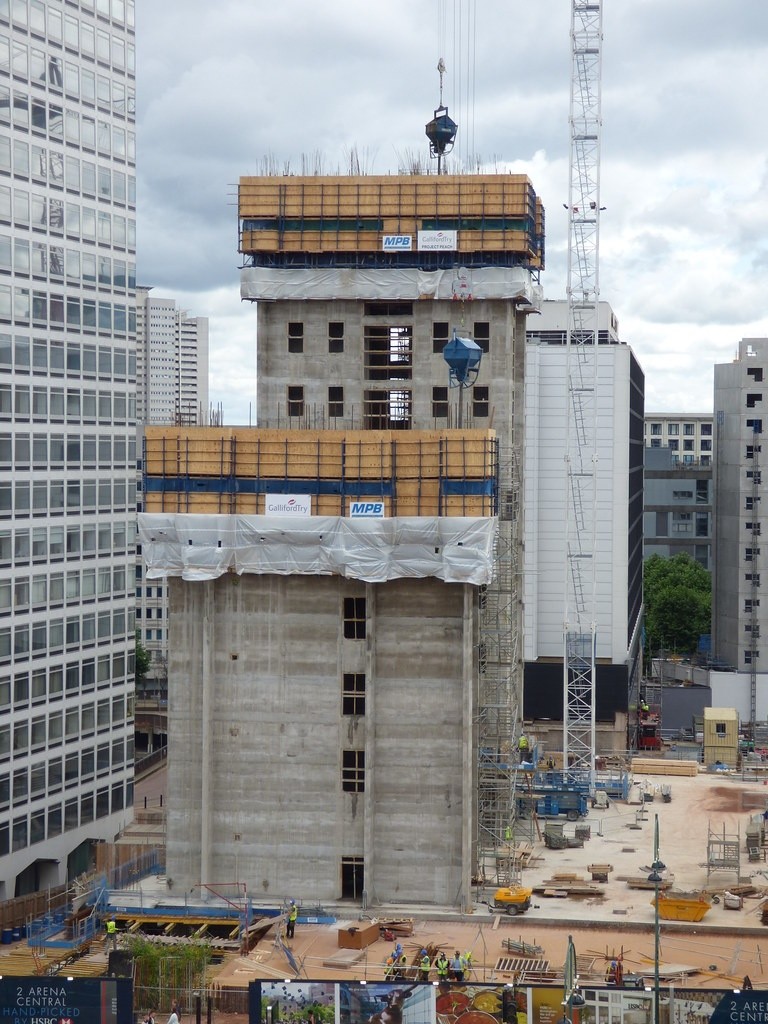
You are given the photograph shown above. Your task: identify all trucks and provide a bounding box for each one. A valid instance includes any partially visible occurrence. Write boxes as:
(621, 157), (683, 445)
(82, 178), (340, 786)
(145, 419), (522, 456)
(513, 786), (590, 823)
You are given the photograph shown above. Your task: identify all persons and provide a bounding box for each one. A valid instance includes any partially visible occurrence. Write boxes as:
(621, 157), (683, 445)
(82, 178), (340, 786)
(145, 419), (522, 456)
(547, 755), (556, 769)
(384, 943), (407, 980)
(104, 915), (119, 955)
(308, 1010), (323, 1024)
(519, 734), (529, 752)
(167, 999), (181, 1024)
(415, 949), (431, 981)
(605, 961), (617, 985)
(435, 951), (467, 982)
(142, 1010), (157, 1024)
(286, 899), (297, 939)
(641, 700), (649, 711)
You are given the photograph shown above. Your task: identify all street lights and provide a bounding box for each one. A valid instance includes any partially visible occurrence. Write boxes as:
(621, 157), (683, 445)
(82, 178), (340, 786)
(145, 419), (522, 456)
(647, 813), (667, 1024)
(555, 934), (587, 1024)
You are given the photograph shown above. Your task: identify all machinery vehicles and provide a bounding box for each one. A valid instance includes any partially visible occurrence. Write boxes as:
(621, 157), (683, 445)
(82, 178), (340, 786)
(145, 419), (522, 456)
(487, 881), (533, 916)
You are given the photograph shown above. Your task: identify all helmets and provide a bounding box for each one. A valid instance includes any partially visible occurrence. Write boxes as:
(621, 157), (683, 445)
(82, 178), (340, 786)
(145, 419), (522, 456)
(420, 950), (427, 955)
(440, 952), (445, 956)
(290, 899), (296, 903)
(397, 949), (402, 954)
(397, 944), (402, 949)
(611, 962), (617, 967)
(391, 952), (398, 957)
(454, 951), (460, 955)
(387, 958), (393, 964)
(111, 916), (116, 921)
(521, 734), (524, 736)
